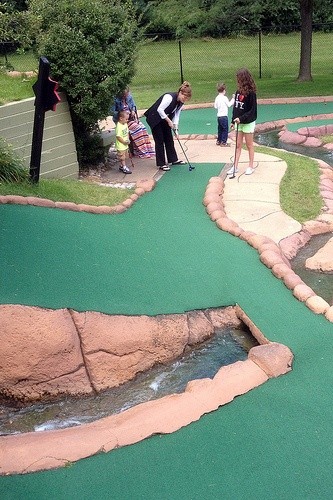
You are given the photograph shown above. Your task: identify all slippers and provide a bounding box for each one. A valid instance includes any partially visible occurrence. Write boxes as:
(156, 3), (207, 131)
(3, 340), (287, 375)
(159, 165), (171, 170)
(172, 161), (186, 165)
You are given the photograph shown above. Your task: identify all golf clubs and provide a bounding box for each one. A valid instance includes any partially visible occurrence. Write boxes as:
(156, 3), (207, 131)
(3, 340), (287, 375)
(173, 129), (195, 169)
(229, 124), (238, 180)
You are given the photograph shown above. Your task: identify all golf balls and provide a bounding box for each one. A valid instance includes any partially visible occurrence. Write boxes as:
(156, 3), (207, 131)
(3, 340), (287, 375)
(189, 168), (192, 171)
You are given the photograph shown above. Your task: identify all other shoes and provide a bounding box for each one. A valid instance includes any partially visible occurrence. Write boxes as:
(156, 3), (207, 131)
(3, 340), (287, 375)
(227, 167), (238, 175)
(245, 167), (255, 175)
(216, 141), (221, 144)
(221, 142), (230, 146)
(123, 167), (132, 174)
(119, 166), (123, 171)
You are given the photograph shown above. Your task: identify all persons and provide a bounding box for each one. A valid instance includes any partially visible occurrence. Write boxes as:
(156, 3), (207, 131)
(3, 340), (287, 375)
(214, 84), (236, 147)
(110, 85), (155, 159)
(144, 81), (192, 171)
(115, 109), (133, 175)
(227, 68), (257, 175)
